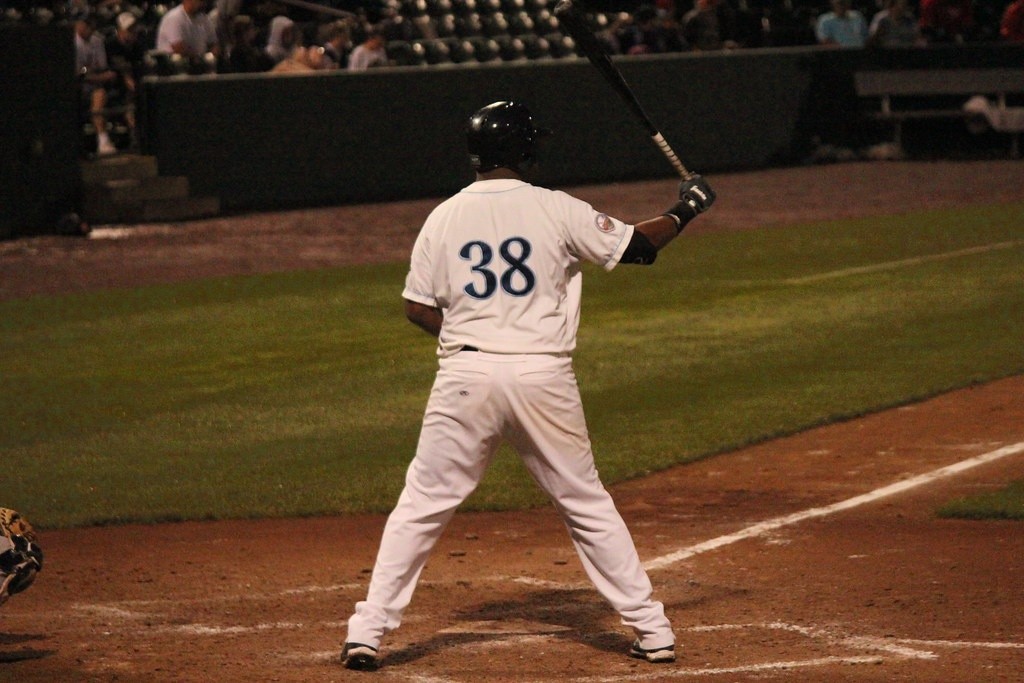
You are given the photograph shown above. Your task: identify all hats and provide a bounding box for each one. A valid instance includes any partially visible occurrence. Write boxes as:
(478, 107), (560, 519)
(114, 10), (138, 32)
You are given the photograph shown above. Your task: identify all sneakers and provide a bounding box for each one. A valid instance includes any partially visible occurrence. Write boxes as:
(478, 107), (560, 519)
(630, 636), (675, 662)
(340, 641), (377, 668)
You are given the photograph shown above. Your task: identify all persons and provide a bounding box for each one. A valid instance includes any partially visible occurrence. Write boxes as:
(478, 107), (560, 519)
(73, 0), (394, 160)
(626, 0), (1024, 54)
(340, 99), (716, 669)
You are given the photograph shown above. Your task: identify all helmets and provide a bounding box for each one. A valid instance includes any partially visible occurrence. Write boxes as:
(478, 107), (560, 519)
(466, 101), (553, 173)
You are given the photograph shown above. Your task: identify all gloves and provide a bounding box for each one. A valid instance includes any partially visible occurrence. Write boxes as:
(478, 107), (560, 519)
(660, 171), (718, 234)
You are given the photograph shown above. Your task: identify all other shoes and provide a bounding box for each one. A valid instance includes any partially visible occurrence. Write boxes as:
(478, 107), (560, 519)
(97, 141), (116, 156)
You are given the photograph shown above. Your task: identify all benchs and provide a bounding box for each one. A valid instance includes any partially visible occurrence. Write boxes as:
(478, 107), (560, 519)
(855, 63), (1024, 160)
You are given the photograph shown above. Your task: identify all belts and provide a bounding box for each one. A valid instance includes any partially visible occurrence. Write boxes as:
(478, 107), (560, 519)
(460, 344), (479, 352)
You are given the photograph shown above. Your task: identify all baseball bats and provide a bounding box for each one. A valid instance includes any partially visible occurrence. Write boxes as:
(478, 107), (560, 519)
(552, 2), (692, 183)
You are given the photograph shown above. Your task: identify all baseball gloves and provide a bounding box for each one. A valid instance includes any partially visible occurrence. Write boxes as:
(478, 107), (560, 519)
(0, 501), (42, 606)
